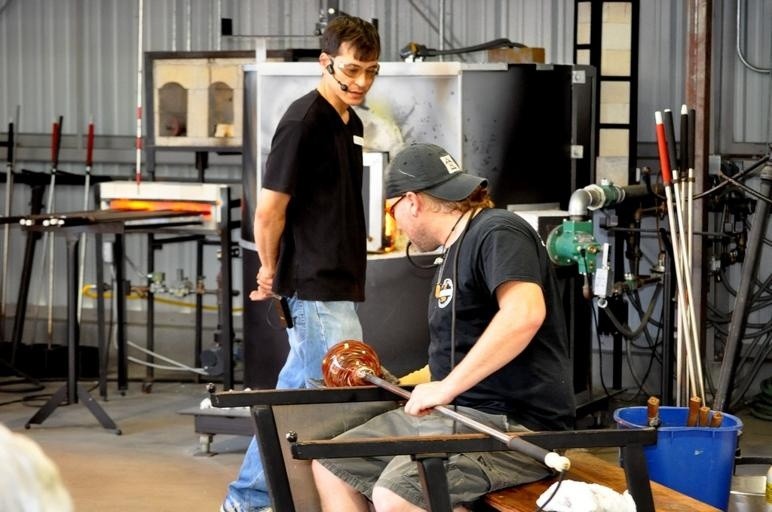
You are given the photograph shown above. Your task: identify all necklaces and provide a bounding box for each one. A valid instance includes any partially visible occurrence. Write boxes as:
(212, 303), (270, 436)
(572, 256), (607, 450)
(432, 207), (477, 299)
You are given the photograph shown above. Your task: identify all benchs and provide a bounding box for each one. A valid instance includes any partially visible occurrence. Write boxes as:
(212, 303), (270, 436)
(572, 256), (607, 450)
(484, 449), (718, 512)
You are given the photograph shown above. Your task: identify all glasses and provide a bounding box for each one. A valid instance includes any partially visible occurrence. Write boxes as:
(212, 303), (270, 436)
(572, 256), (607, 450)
(383, 194), (407, 221)
(333, 59), (382, 81)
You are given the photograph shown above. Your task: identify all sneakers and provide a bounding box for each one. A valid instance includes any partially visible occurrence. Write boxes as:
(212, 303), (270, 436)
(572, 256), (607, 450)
(219, 494), (274, 512)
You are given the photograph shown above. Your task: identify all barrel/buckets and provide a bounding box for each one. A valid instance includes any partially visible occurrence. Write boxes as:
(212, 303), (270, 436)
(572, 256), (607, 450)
(613, 404), (745, 512)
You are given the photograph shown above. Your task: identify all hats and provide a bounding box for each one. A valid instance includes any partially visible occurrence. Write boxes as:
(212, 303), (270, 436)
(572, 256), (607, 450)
(384, 143), (489, 202)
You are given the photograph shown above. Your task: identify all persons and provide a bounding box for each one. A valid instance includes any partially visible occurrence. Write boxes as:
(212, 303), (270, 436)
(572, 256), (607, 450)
(311, 143), (575, 511)
(219, 14), (381, 512)
